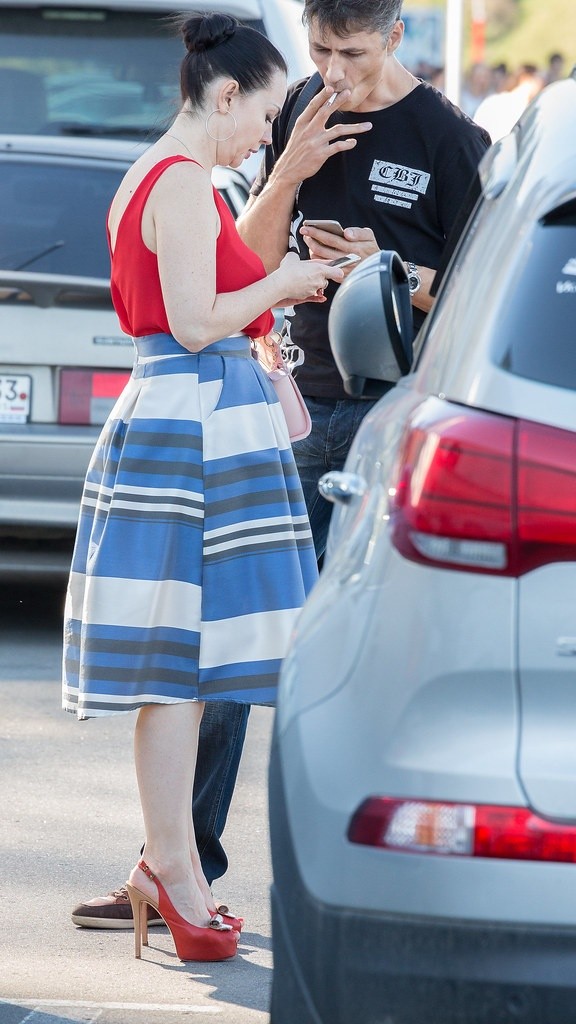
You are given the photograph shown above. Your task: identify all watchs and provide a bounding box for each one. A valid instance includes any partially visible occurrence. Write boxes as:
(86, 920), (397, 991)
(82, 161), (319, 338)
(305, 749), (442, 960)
(405, 261), (421, 300)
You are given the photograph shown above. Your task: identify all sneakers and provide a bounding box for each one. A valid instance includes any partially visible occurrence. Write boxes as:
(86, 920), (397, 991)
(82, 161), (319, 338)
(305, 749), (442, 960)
(71, 885), (167, 928)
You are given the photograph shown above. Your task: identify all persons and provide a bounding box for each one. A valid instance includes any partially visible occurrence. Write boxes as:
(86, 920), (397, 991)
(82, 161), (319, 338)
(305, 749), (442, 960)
(58, 12), (350, 964)
(417, 51), (572, 139)
(69, 0), (502, 933)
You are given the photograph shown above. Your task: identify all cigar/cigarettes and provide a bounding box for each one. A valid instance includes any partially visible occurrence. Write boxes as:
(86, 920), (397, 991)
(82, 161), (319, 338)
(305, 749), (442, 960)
(326, 92), (338, 106)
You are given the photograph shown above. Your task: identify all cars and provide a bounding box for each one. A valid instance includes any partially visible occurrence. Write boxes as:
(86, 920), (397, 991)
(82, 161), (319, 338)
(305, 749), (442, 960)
(1, 123), (254, 596)
(257, 72), (576, 1024)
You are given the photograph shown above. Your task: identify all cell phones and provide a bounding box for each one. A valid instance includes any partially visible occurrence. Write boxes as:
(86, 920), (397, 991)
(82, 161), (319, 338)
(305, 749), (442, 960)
(326, 253), (362, 268)
(303, 219), (344, 238)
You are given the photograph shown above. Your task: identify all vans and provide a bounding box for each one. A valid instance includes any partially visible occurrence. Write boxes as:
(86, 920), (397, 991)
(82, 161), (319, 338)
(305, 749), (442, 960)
(0, 0), (310, 187)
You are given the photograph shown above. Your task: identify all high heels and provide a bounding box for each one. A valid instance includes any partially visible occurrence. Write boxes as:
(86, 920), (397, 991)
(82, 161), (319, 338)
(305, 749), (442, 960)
(140, 886), (244, 946)
(126, 858), (240, 961)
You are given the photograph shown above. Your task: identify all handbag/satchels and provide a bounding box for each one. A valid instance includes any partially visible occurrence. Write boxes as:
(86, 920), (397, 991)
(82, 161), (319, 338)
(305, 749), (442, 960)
(257, 331), (312, 443)
